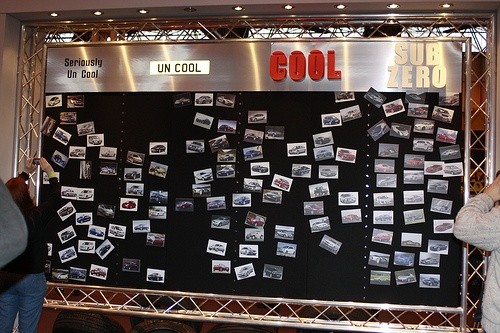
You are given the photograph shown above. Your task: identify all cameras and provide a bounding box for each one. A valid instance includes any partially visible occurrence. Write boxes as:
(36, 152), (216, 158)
(32, 158), (41, 165)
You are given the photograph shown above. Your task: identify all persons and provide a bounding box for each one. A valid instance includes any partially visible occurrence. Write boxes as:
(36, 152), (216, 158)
(452, 171), (500, 333)
(0, 156), (60, 333)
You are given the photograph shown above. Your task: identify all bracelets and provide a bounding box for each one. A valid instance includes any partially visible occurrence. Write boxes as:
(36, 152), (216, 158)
(48, 172), (56, 180)
